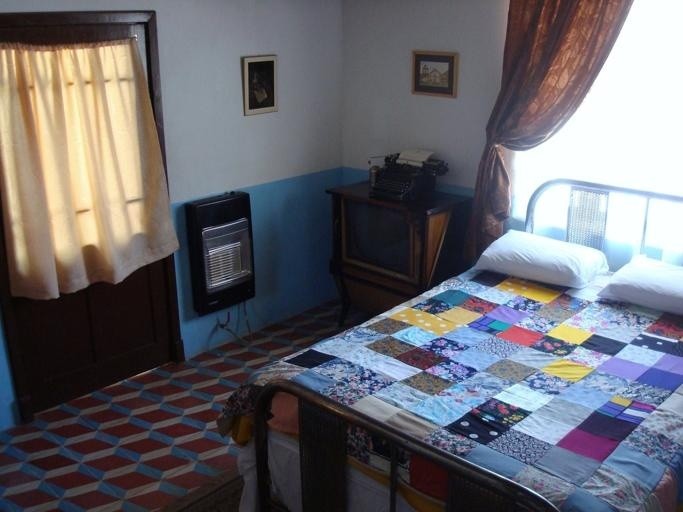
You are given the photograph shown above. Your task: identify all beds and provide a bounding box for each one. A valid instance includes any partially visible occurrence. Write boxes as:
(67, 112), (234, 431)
(217, 178), (682, 511)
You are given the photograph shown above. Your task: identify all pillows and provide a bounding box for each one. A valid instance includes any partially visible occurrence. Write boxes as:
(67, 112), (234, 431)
(473, 228), (682, 317)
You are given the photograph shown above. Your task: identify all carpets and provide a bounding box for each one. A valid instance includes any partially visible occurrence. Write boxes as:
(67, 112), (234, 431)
(177, 465), (291, 512)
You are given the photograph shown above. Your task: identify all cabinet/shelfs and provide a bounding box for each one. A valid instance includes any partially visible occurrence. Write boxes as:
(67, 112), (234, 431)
(324, 182), (474, 327)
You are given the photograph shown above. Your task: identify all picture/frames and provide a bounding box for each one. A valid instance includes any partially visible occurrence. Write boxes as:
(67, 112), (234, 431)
(243, 53), (279, 117)
(411, 49), (460, 99)
